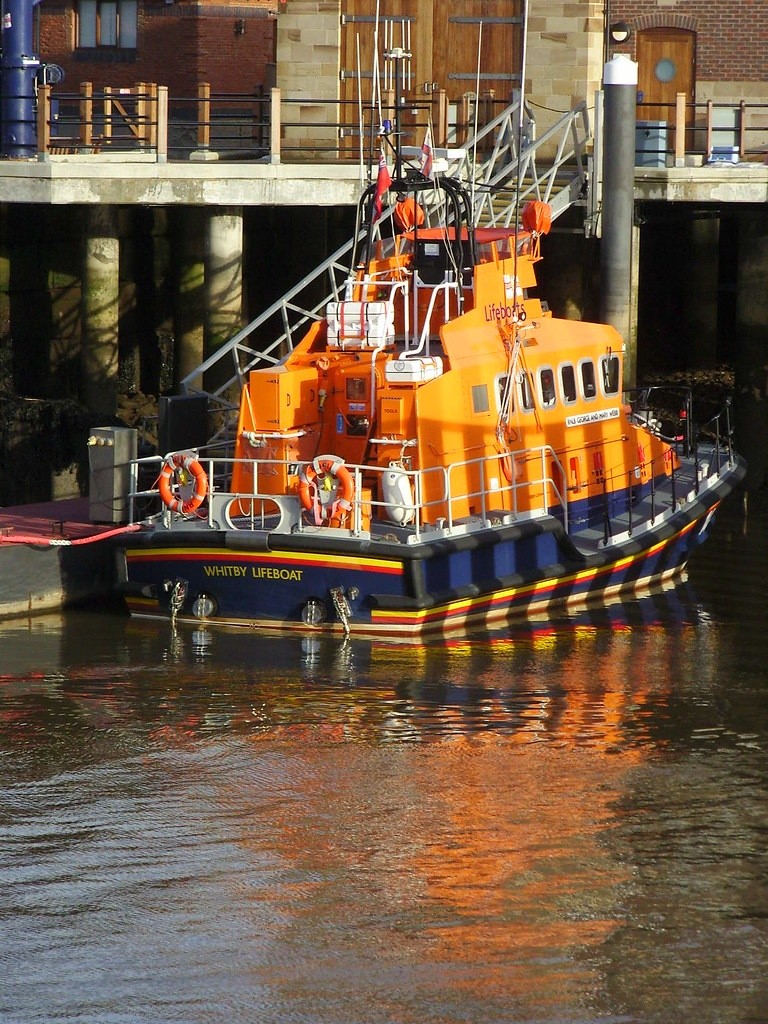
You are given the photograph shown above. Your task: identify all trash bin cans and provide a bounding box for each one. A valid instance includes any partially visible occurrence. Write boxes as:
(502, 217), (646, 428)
(635, 119), (668, 167)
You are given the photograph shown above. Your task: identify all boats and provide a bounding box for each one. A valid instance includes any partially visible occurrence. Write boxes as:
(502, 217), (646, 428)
(118, 0), (750, 646)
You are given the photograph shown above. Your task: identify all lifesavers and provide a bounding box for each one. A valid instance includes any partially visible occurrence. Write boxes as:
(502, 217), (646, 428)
(158, 453), (208, 514)
(297, 454), (356, 519)
